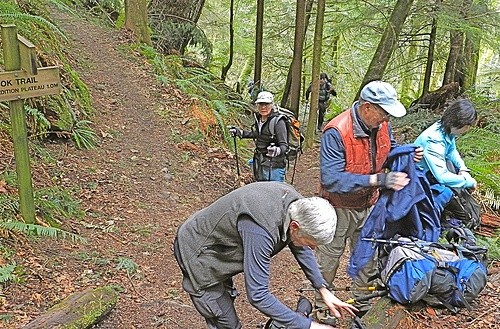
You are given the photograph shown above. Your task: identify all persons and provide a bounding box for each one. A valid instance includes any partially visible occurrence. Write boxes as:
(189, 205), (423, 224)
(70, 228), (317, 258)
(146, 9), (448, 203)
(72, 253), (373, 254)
(314, 79), (423, 329)
(226, 92), (287, 182)
(173, 180), (359, 329)
(412, 99), (482, 230)
(305, 73), (337, 135)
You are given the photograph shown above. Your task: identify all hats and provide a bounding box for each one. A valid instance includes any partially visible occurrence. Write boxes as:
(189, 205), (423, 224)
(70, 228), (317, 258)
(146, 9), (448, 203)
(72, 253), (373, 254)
(360, 81), (406, 118)
(255, 91), (274, 103)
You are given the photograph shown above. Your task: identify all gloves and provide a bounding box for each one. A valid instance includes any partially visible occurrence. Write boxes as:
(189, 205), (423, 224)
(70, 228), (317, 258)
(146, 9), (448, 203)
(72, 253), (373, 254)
(227, 125), (243, 139)
(266, 146), (280, 157)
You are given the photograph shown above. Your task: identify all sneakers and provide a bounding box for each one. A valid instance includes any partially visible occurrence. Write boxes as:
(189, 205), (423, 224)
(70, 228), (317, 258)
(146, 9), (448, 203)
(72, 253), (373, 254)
(313, 301), (337, 328)
(349, 291), (371, 316)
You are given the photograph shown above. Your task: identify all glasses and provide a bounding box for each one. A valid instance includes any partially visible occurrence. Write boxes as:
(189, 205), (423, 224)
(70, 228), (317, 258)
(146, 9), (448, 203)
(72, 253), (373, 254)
(372, 105), (391, 120)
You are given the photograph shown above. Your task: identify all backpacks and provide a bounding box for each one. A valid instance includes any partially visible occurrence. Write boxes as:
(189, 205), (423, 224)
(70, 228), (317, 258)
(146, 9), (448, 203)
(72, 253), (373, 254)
(319, 80), (331, 101)
(264, 295), (366, 329)
(254, 106), (304, 161)
(378, 158), (489, 313)
